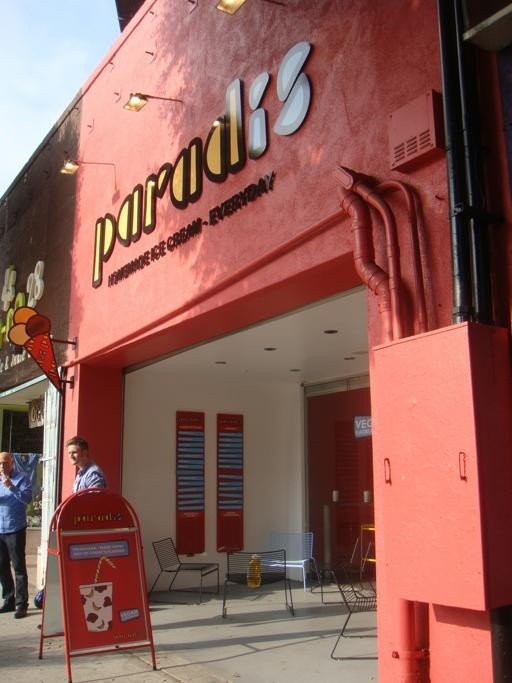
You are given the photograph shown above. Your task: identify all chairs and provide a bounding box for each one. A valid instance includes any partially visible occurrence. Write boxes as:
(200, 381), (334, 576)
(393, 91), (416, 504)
(222, 549), (294, 618)
(330, 563), (378, 660)
(258, 532), (323, 594)
(147, 537), (220, 605)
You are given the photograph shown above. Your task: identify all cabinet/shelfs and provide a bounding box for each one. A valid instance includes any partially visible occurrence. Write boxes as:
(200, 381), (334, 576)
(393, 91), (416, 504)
(360, 524), (376, 577)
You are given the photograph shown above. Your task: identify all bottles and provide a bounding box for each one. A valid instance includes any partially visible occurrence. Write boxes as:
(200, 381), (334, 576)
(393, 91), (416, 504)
(247, 553), (261, 588)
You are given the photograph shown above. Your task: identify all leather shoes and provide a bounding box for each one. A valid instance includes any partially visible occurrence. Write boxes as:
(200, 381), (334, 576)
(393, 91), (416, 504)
(0, 603), (27, 618)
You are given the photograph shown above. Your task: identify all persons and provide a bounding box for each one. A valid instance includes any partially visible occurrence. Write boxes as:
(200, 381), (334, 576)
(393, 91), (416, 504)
(37, 436), (109, 631)
(0, 452), (33, 620)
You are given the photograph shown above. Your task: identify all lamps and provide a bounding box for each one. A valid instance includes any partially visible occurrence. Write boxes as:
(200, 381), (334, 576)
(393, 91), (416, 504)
(214, 0), (283, 16)
(58, 159), (115, 176)
(122, 93), (183, 113)
